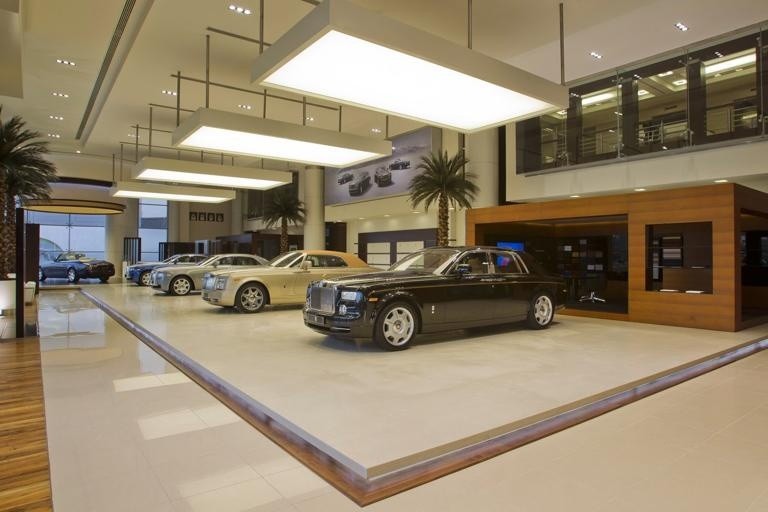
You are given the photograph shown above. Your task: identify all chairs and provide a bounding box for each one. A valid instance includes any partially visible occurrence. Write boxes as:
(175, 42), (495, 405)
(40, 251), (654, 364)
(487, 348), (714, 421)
(467, 258), (483, 273)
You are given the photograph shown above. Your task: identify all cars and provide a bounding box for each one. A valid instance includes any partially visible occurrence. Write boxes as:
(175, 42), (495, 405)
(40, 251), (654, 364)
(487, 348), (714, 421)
(122, 246), (387, 315)
(336, 156), (410, 196)
(299, 243), (571, 351)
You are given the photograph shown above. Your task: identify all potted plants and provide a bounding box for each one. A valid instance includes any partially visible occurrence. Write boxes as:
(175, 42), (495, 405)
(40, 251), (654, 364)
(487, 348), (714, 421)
(0, 104), (58, 341)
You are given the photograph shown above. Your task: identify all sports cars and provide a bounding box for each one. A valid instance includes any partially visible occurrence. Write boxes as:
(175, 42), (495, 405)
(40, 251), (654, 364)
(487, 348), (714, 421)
(38, 251), (116, 284)
